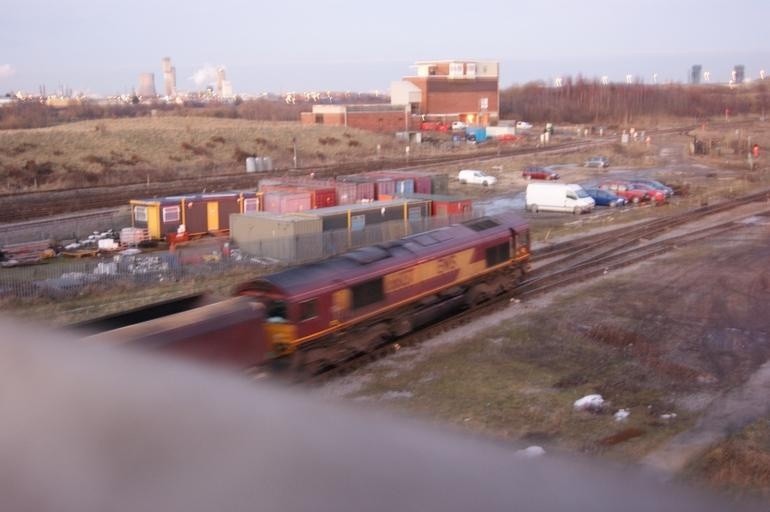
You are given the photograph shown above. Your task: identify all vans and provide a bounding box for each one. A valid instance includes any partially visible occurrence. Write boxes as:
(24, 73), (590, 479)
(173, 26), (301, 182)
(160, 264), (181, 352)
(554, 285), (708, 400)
(525, 182), (596, 216)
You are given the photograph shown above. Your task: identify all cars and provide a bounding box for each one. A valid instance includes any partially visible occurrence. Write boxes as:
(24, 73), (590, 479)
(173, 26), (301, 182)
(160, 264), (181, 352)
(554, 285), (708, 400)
(522, 166), (560, 180)
(585, 179), (674, 208)
(458, 170), (497, 186)
(582, 155), (609, 168)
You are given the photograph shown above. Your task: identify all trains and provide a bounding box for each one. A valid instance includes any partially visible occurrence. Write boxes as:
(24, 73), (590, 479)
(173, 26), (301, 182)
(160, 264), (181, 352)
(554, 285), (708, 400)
(85, 211), (533, 380)
